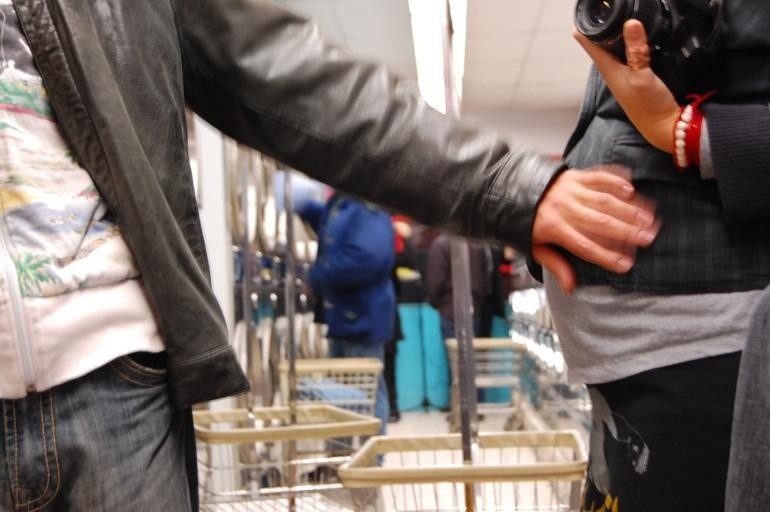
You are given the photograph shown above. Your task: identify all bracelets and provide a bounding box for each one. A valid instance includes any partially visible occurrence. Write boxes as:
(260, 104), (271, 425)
(672, 93), (715, 171)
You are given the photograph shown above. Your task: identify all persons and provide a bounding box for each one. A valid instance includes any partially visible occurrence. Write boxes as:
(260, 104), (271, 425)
(296, 188), (394, 488)
(424, 229), (489, 422)
(3, 3), (660, 512)
(532, 2), (770, 509)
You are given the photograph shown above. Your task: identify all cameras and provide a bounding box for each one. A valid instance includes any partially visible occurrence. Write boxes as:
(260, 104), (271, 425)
(575, 0), (723, 66)
